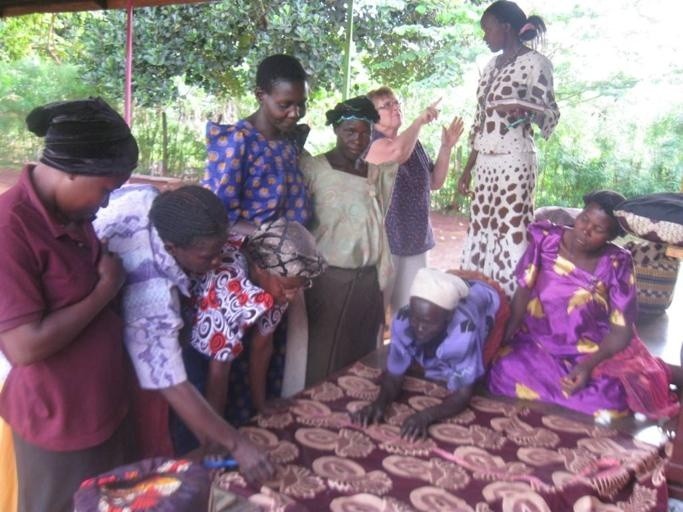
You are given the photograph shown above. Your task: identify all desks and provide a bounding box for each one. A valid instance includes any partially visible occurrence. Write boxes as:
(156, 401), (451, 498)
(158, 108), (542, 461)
(124, 336), (681, 512)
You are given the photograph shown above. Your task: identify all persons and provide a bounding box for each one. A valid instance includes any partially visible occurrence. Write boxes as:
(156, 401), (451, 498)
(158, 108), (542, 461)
(177, 216), (327, 453)
(488, 192), (683, 422)
(92, 182), (276, 481)
(457, 1), (561, 300)
(298, 95), (394, 389)
(349, 267), (512, 443)
(362, 86), (464, 344)
(202, 54), (317, 429)
(0, 95), (143, 511)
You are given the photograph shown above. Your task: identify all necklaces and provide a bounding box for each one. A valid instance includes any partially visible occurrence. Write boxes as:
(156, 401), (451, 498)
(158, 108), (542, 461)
(569, 238), (574, 263)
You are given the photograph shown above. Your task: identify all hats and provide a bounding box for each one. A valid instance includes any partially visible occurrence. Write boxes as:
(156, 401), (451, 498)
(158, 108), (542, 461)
(410, 267), (469, 311)
(246, 218), (328, 278)
(27, 97), (138, 176)
(583, 191), (627, 237)
(327, 96), (378, 126)
